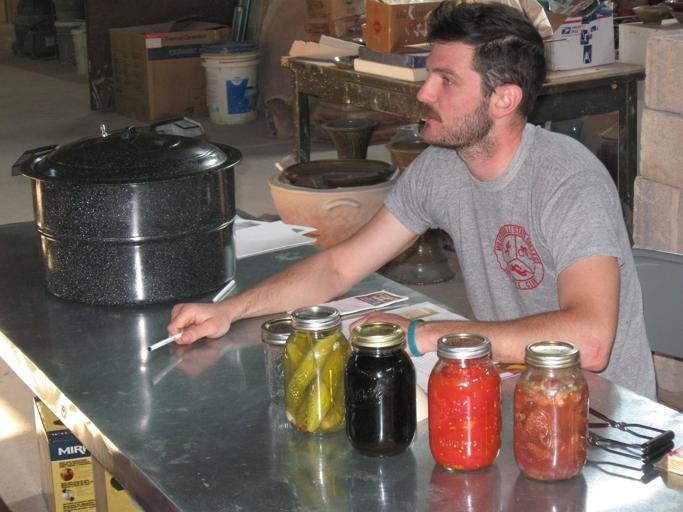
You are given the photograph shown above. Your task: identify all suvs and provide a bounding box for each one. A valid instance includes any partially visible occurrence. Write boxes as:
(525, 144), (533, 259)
(199, 50), (260, 125)
(42, 21), (88, 76)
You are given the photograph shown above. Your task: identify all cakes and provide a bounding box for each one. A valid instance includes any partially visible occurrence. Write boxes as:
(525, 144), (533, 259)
(344, 323), (416, 456)
(283, 303), (354, 433)
(428, 332), (501, 471)
(513, 341), (590, 479)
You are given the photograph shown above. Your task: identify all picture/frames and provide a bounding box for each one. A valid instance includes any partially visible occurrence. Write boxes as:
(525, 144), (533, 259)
(1, 209), (683, 512)
(281, 56), (645, 247)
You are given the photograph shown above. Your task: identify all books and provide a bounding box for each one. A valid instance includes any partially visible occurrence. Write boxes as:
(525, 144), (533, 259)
(107, 18), (234, 125)
(539, 1), (617, 72)
(364, 0), (447, 59)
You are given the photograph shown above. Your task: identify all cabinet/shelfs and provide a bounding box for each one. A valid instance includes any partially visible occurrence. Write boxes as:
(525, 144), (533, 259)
(343, 321), (418, 459)
(511, 339), (591, 482)
(426, 331), (504, 473)
(260, 315), (294, 403)
(281, 304), (352, 436)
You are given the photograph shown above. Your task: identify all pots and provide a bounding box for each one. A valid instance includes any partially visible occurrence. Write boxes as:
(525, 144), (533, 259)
(10, 124), (245, 309)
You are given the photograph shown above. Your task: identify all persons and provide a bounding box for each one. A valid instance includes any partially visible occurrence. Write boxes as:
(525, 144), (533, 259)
(164, 0), (660, 403)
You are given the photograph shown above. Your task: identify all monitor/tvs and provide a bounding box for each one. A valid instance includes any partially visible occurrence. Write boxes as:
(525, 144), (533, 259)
(334, 300), (521, 398)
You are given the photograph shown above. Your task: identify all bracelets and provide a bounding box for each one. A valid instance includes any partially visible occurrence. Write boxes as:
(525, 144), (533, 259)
(632, 5), (673, 25)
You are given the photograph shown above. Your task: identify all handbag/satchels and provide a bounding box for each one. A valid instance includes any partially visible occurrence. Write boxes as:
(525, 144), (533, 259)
(406, 318), (426, 358)
